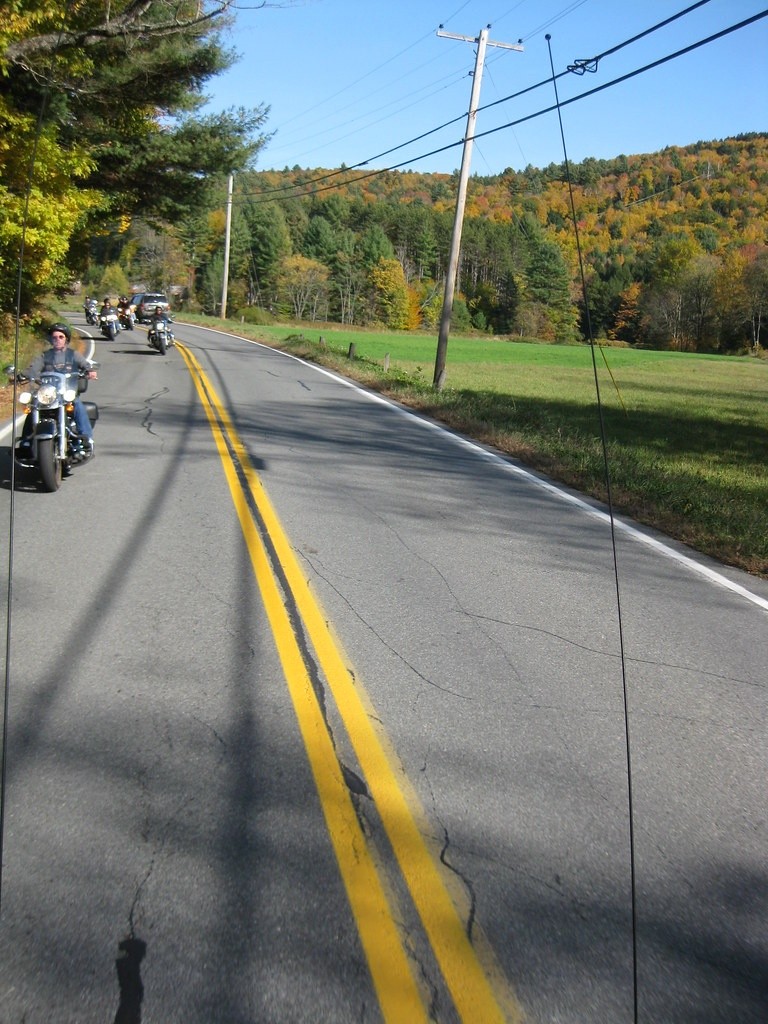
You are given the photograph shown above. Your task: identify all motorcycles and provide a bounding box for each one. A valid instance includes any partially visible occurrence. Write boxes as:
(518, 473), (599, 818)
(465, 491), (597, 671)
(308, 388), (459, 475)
(116, 304), (139, 330)
(1, 357), (101, 492)
(82, 303), (100, 326)
(95, 309), (121, 341)
(143, 314), (176, 355)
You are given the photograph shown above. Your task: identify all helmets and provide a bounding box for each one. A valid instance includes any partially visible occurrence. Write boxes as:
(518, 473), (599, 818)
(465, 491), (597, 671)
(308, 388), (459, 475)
(104, 298), (110, 303)
(49, 322), (70, 343)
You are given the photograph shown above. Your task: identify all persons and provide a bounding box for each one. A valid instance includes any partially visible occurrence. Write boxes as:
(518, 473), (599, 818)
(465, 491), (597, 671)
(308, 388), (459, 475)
(83, 296), (119, 334)
(117, 297), (135, 327)
(7, 322), (94, 456)
(146, 307), (175, 347)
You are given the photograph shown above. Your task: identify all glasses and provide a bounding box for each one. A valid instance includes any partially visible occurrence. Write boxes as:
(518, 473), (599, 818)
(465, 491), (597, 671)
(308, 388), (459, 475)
(52, 335), (65, 340)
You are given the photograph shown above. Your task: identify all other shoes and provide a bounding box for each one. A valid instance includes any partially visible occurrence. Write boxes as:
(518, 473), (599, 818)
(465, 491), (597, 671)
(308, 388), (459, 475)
(20, 440), (32, 448)
(85, 438), (94, 452)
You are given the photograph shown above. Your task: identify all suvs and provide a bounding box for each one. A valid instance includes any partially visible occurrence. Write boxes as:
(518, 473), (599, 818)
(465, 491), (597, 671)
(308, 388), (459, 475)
(130, 292), (168, 324)
